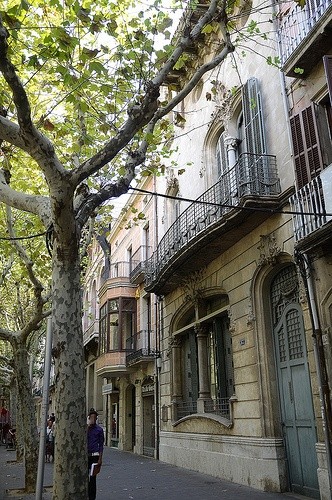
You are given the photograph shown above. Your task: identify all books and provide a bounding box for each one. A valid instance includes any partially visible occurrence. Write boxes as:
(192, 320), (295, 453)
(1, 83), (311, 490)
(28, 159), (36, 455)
(89, 463), (100, 477)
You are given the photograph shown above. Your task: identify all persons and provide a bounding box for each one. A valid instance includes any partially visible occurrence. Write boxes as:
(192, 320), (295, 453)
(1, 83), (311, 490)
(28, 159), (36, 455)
(106, 418), (115, 437)
(87, 412), (104, 500)
(3, 413), (56, 459)
(87, 408), (99, 425)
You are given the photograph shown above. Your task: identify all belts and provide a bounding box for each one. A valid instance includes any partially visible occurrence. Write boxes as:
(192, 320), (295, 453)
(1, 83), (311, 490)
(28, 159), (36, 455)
(88, 452), (99, 456)
